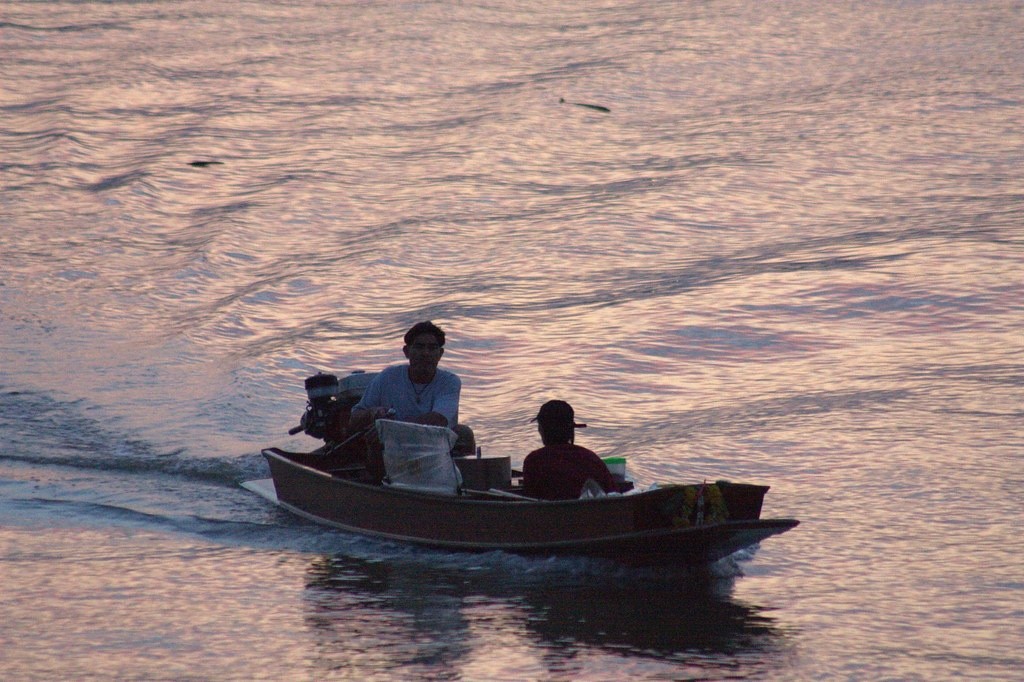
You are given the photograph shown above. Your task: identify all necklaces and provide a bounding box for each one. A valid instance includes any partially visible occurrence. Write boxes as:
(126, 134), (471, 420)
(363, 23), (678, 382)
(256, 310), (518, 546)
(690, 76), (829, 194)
(407, 367), (436, 404)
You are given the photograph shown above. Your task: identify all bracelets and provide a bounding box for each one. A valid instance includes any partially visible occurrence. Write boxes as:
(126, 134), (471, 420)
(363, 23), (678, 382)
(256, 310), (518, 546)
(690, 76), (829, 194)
(373, 407), (378, 418)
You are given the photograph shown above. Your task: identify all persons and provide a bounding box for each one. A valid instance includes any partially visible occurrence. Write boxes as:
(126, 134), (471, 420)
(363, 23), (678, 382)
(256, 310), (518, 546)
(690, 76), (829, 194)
(523, 400), (634, 501)
(348, 321), (475, 456)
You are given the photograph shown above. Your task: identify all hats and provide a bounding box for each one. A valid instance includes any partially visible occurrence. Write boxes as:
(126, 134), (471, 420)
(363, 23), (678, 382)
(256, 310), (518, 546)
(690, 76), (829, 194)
(531, 400), (586, 434)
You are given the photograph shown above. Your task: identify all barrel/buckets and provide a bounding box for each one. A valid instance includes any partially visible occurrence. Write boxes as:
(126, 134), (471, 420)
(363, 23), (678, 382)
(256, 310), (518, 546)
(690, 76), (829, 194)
(601, 456), (626, 482)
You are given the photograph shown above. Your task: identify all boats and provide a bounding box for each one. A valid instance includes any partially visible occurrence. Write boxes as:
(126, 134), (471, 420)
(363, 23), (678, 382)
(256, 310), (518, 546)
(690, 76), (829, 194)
(260, 445), (799, 571)
